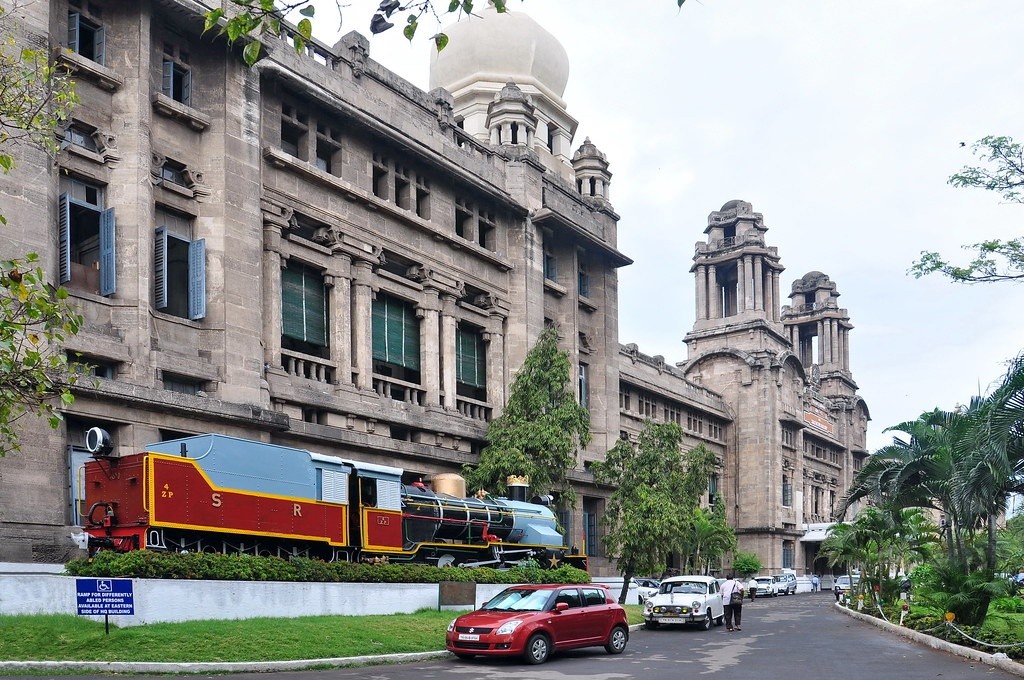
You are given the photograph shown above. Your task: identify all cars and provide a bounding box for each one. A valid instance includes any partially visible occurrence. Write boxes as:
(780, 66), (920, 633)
(748, 577), (778, 598)
(643, 575), (725, 631)
(716, 579), (727, 587)
(633, 578), (660, 605)
(445, 584), (629, 665)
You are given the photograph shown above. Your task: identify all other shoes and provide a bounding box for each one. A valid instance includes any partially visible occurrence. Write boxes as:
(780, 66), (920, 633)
(729, 628), (734, 632)
(752, 600), (754, 602)
(737, 628), (741, 630)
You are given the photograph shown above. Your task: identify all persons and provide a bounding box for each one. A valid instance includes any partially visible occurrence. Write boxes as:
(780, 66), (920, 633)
(748, 576), (758, 602)
(811, 575), (818, 594)
(720, 573), (745, 632)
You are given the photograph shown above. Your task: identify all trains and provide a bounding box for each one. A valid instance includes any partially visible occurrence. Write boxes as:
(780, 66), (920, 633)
(71, 426), (588, 574)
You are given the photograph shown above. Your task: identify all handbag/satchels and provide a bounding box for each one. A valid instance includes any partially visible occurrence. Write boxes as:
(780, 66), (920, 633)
(730, 580), (743, 606)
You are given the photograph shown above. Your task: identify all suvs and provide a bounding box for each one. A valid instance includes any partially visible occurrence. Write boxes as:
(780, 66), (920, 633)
(834, 575), (861, 600)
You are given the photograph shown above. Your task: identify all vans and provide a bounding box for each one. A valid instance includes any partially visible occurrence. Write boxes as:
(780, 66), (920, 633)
(773, 573), (797, 595)
